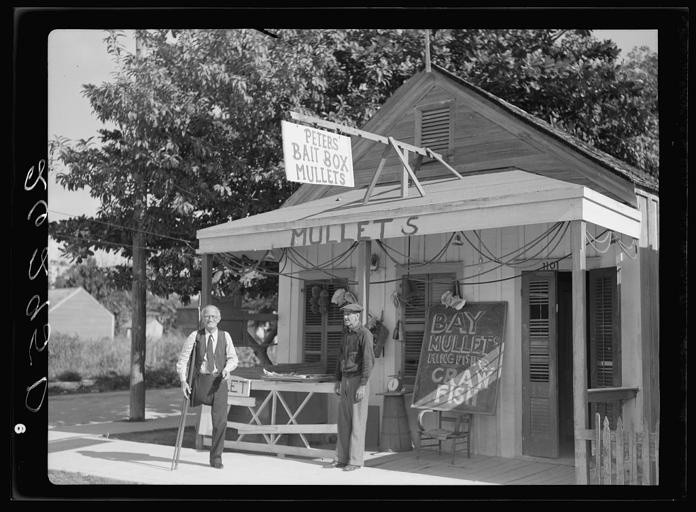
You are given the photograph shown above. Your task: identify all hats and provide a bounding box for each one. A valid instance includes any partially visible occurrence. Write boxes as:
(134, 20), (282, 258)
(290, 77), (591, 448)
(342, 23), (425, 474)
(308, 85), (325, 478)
(338, 303), (363, 313)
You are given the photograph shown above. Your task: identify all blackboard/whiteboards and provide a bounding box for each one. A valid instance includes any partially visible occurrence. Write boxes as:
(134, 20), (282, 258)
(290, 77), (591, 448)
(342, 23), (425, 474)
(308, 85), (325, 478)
(410, 301), (508, 416)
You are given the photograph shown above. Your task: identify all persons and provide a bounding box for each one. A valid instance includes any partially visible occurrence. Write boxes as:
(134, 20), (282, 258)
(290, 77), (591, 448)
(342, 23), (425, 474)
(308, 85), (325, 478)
(321, 301), (376, 473)
(176, 304), (239, 469)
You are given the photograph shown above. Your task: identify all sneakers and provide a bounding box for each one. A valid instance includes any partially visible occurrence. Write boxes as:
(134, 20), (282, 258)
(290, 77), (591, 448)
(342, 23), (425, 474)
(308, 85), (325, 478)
(323, 460), (361, 470)
(213, 463), (223, 469)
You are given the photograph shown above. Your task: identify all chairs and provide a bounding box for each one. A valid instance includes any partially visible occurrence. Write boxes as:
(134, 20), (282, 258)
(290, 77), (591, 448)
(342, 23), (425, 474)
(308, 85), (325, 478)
(413, 406), (473, 465)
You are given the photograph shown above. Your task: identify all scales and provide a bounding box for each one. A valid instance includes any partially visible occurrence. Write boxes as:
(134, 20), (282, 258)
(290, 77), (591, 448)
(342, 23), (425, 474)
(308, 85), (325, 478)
(386, 374), (405, 392)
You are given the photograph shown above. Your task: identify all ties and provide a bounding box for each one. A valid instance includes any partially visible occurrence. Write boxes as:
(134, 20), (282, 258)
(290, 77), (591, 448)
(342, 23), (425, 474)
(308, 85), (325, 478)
(206, 335), (214, 372)
